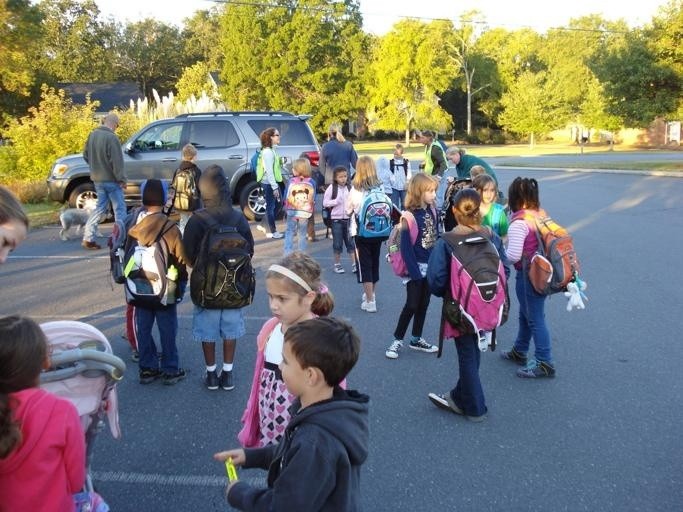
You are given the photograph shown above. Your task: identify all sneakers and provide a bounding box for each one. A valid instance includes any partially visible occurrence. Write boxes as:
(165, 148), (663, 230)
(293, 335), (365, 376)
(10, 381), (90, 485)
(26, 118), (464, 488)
(361, 299), (377, 313)
(499, 350), (529, 365)
(159, 369), (187, 386)
(217, 368), (237, 391)
(308, 238), (319, 243)
(80, 240), (101, 250)
(483, 328), (498, 346)
(361, 290), (376, 302)
(408, 338), (439, 353)
(516, 358), (557, 378)
(256, 224), (268, 234)
(131, 352), (140, 363)
(428, 390), (453, 411)
(333, 263), (346, 274)
(203, 370), (220, 390)
(384, 338), (405, 359)
(351, 263), (357, 273)
(140, 367), (163, 384)
(266, 231), (284, 239)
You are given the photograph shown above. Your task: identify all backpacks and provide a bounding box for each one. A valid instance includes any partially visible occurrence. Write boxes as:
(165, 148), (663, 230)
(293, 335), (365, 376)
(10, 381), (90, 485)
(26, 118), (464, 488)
(527, 218), (581, 296)
(140, 178), (169, 205)
(385, 222), (410, 278)
(439, 234), (511, 333)
(171, 172), (201, 212)
(321, 205), (333, 229)
(359, 190), (395, 242)
(250, 148), (261, 180)
(286, 181), (314, 220)
(123, 236), (179, 308)
(438, 140), (447, 152)
(106, 213), (136, 285)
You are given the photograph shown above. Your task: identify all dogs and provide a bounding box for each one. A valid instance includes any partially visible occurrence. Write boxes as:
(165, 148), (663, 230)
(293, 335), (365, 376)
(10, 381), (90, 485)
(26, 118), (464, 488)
(58, 200), (113, 241)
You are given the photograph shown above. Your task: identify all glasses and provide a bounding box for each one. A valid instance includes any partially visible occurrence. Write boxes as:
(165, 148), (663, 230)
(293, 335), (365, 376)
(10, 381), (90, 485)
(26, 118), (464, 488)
(271, 133), (280, 137)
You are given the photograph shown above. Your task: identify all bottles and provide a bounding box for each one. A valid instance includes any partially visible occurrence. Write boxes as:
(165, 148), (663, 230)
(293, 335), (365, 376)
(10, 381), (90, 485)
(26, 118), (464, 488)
(389, 244), (398, 253)
(167, 265), (178, 305)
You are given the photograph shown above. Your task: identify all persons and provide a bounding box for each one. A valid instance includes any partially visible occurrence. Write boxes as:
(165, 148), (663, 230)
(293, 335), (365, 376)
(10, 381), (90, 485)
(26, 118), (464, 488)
(171, 142), (202, 235)
(120, 179), (168, 361)
(182, 164), (255, 391)
(123, 178), (186, 386)
(0, 314), (86, 512)
(254, 127), (554, 421)
(0, 183), (32, 266)
(81, 113), (128, 250)
(213, 252), (370, 512)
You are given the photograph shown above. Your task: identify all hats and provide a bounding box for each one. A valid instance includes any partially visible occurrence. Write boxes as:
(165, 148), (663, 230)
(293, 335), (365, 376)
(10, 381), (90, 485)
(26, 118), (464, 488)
(190, 223), (257, 311)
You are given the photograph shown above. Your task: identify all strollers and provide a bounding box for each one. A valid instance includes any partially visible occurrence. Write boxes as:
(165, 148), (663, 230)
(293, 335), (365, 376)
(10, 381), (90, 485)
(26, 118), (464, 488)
(437, 178), (471, 235)
(26, 316), (125, 506)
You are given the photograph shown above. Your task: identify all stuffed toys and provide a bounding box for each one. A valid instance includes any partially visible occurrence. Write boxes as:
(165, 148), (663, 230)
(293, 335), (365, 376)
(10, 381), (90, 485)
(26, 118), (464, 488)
(573, 271), (588, 302)
(563, 283), (585, 312)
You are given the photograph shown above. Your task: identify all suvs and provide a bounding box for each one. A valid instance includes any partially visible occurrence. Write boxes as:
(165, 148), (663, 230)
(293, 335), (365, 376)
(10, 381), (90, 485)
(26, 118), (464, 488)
(46, 110), (324, 219)
